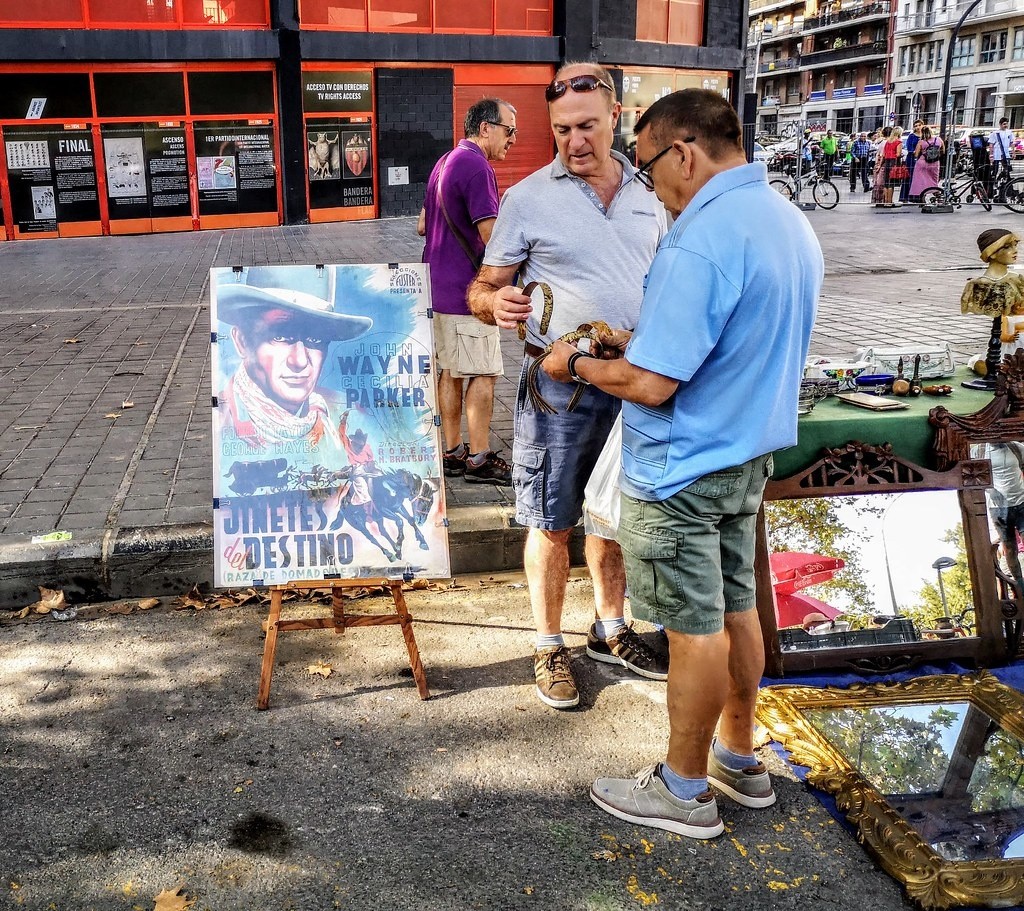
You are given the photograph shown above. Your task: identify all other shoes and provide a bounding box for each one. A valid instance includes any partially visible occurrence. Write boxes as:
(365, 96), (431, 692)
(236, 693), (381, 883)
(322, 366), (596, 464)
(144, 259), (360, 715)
(850, 186), (855, 192)
(864, 186), (873, 192)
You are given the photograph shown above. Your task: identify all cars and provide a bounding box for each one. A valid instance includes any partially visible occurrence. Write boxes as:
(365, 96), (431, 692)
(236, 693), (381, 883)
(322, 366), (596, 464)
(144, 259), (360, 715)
(753, 125), (1024, 176)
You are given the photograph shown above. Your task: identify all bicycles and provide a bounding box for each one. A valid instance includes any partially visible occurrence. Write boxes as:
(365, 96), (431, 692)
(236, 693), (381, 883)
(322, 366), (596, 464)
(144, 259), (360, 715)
(1000, 175), (1024, 214)
(919, 163), (993, 212)
(768, 152), (840, 210)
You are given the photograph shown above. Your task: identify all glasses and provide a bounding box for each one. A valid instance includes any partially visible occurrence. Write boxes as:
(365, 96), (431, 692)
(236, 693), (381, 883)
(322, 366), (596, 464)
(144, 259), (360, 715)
(545, 74), (614, 100)
(483, 119), (518, 137)
(634, 136), (695, 188)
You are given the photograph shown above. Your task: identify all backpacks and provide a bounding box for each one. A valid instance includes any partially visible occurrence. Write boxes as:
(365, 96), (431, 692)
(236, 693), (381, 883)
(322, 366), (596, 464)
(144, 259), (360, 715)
(921, 136), (940, 163)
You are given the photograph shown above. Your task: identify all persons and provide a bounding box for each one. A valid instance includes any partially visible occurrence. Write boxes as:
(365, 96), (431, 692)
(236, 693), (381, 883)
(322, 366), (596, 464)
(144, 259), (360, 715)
(418, 97), (512, 486)
(820, 129), (840, 177)
(539, 87), (822, 839)
(802, 613), (831, 633)
(960, 228), (1024, 316)
(846, 130), (880, 192)
(872, 119), (945, 208)
(799, 128), (813, 170)
(469, 62), (667, 711)
(988, 117), (1017, 177)
(968, 438), (1024, 582)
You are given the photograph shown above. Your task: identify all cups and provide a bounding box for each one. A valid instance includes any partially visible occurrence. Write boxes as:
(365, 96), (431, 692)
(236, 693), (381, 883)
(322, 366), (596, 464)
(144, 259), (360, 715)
(216, 166), (234, 187)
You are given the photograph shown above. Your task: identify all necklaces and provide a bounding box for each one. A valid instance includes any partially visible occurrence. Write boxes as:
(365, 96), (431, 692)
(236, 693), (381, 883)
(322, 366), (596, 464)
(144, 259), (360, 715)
(981, 270), (1009, 281)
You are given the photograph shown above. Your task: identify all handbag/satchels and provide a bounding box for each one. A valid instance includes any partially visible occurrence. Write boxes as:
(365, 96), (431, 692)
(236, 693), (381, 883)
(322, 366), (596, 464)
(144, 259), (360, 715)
(889, 164), (910, 179)
(582, 404), (623, 538)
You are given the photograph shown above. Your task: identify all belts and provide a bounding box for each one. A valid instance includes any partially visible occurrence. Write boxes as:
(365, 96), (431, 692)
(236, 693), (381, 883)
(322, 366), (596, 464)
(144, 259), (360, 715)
(523, 342), (544, 359)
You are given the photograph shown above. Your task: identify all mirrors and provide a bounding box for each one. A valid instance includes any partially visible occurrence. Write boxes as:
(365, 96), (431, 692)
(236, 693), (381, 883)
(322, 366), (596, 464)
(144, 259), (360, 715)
(754, 441), (1011, 678)
(748, 668), (1024, 911)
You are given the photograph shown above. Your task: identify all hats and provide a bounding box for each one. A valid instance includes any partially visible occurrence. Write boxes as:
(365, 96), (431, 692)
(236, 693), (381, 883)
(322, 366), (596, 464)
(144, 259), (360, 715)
(803, 613), (830, 629)
(804, 128), (811, 133)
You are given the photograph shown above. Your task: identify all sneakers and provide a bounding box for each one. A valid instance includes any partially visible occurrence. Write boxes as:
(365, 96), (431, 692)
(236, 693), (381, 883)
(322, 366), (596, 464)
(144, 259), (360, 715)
(442, 445), (471, 475)
(707, 735), (776, 809)
(590, 763), (724, 838)
(586, 619), (669, 680)
(534, 643), (579, 708)
(464, 450), (513, 485)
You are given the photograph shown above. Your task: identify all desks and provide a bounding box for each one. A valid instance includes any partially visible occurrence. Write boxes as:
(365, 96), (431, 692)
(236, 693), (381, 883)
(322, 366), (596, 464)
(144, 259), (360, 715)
(767, 363), (1010, 481)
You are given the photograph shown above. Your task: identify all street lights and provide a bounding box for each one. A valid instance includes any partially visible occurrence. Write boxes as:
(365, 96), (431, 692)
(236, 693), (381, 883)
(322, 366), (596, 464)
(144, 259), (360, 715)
(932, 556), (959, 626)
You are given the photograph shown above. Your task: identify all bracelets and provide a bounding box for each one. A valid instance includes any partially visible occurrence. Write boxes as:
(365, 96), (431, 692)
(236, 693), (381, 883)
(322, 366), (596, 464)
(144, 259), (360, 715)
(567, 351), (596, 385)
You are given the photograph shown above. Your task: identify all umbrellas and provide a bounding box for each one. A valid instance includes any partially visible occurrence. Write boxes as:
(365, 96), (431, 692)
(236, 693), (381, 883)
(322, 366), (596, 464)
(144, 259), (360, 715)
(776, 592), (845, 629)
(770, 552), (844, 595)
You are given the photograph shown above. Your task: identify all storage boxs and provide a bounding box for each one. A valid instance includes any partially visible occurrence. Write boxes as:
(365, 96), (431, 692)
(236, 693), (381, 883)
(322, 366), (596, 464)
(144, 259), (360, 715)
(778, 617), (918, 650)
(862, 340), (950, 379)
(803, 360), (871, 389)
